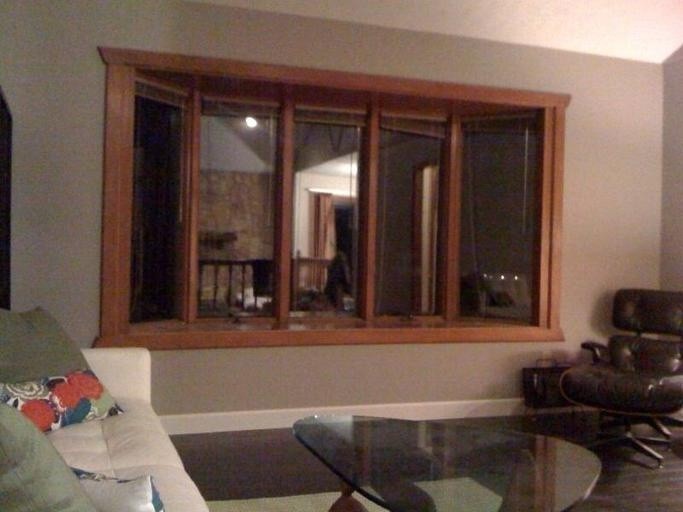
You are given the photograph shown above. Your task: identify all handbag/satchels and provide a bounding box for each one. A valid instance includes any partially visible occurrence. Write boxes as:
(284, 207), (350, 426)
(522, 357), (572, 408)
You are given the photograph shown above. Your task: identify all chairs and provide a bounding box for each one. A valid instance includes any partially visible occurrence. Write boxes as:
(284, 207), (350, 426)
(559, 286), (683, 470)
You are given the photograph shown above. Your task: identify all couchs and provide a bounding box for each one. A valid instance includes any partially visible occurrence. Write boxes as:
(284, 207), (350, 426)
(0, 346), (210, 511)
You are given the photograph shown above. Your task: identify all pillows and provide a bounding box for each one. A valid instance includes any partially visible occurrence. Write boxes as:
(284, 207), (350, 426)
(0, 306), (162, 511)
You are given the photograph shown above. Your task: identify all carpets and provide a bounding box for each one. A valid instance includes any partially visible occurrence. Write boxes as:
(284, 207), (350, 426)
(203, 476), (503, 511)
